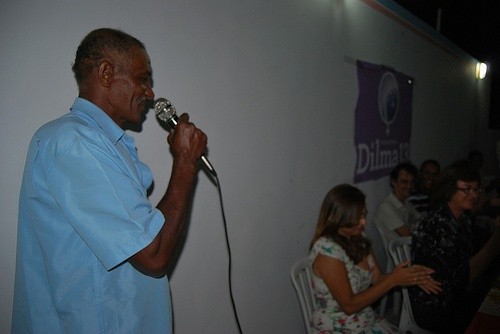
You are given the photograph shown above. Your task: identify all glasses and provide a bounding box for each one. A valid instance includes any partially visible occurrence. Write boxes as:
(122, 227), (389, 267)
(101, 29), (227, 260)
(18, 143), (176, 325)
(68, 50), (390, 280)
(456, 187), (483, 194)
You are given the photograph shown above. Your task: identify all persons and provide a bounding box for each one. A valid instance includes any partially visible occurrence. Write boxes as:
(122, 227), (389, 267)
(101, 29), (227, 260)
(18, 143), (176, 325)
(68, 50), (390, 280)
(379, 159), (500, 334)
(308, 184), (442, 334)
(12, 27), (208, 334)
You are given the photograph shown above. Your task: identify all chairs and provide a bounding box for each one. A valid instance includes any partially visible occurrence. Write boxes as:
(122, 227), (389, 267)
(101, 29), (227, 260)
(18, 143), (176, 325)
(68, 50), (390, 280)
(291, 257), (315, 334)
(374, 219), (428, 334)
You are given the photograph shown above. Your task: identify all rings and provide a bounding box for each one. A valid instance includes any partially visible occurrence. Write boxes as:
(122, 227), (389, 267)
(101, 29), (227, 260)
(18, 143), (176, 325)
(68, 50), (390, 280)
(414, 279), (417, 281)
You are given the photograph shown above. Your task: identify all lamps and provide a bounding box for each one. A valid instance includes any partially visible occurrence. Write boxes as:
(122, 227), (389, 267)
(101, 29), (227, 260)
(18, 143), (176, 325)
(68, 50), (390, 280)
(475, 62), (487, 79)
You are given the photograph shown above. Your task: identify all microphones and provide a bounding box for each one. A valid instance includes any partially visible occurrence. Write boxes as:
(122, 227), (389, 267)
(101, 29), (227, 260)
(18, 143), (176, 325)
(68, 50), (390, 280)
(154, 98), (217, 178)
